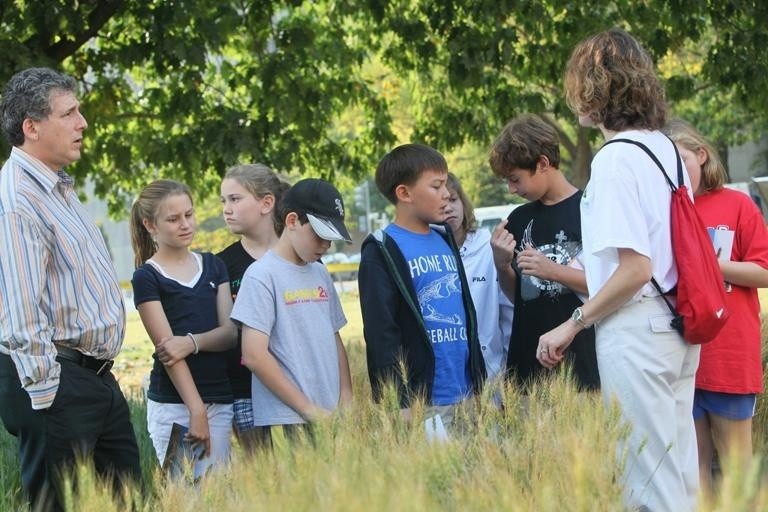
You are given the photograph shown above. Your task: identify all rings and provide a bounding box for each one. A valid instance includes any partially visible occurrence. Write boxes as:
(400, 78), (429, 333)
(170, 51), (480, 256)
(541, 349), (547, 353)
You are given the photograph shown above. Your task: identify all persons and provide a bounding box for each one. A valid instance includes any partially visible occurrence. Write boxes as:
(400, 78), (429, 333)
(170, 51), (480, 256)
(130, 179), (238, 489)
(358, 143), (488, 428)
(535, 30), (701, 511)
(217, 164), (293, 463)
(230, 178), (353, 461)
(445, 172), (515, 408)
(0, 67), (143, 512)
(663, 117), (768, 512)
(490, 114), (600, 395)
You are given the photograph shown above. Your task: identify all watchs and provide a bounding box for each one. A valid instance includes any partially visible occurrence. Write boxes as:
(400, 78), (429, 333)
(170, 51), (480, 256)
(572, 307), (591, 330)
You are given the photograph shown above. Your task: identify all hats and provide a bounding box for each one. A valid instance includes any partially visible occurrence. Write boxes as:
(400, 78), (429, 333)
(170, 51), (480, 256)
(285, 177), (357, 247)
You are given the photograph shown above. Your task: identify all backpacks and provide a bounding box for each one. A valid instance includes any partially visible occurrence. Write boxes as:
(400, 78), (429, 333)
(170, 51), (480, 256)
(594, 130), (732, 346)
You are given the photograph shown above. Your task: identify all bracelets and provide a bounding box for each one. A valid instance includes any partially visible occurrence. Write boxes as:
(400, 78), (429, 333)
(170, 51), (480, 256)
(187, 333), (199, 354)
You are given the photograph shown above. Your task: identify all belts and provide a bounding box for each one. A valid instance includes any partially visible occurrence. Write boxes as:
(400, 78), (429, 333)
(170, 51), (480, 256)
(55, 345), (114, 379)
